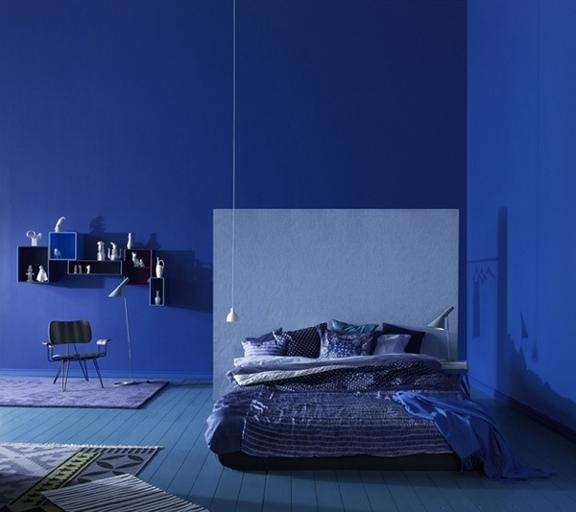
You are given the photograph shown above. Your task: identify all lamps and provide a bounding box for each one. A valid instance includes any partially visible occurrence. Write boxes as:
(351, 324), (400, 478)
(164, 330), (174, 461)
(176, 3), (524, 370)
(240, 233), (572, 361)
(428, 305), (456, 361)
(223, 0), (242, 324)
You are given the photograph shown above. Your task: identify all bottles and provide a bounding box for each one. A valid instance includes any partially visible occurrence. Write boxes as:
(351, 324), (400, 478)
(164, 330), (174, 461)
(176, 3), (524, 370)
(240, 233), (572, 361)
(154, 291), (160, 305)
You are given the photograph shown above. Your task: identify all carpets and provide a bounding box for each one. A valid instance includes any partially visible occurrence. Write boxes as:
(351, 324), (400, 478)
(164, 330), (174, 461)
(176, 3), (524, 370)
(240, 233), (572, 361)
(1, 440), (210, 512)
(0, 376), (169, 411)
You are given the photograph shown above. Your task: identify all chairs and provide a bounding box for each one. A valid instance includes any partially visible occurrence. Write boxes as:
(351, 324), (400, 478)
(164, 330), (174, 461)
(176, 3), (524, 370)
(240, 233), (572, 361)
(39, 319), (112, 393)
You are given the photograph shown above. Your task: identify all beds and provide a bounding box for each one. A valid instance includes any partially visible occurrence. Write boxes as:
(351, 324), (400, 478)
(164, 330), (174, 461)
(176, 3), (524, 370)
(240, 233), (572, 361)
(209, 350), (489, 472)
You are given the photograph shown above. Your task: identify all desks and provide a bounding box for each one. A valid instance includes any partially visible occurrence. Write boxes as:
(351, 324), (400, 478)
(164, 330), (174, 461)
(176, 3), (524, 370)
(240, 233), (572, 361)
(436, 358), (471, 395)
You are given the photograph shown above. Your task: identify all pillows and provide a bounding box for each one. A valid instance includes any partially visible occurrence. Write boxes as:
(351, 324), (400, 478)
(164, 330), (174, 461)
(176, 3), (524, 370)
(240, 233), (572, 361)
(238, 317), (427, 364)
